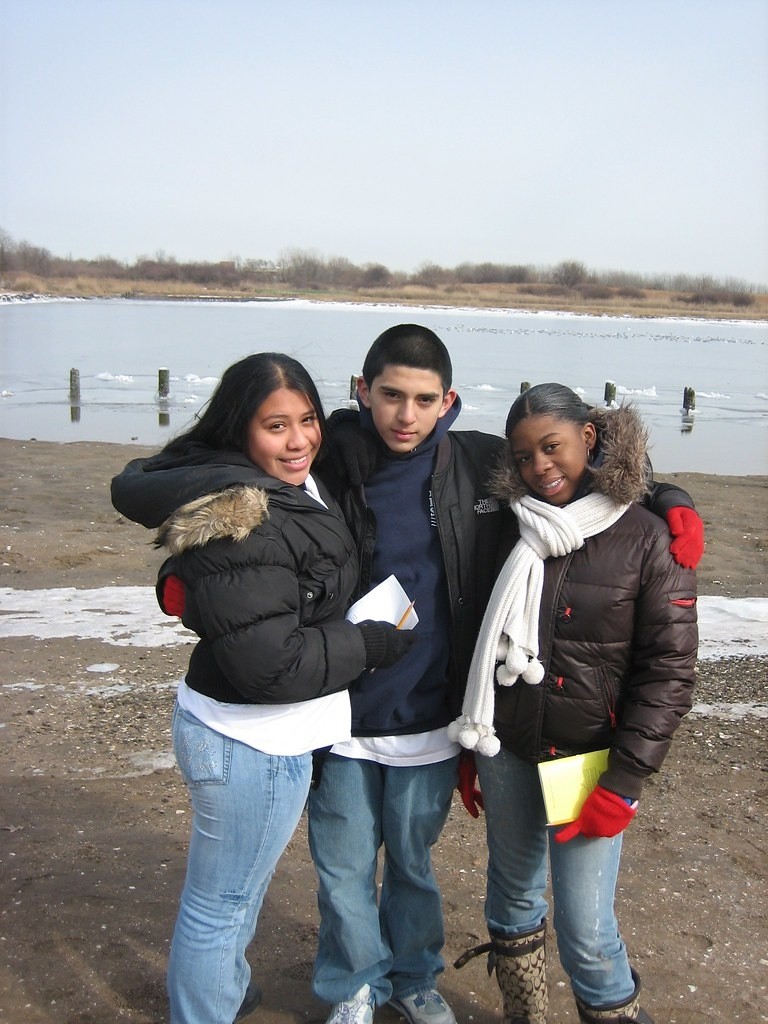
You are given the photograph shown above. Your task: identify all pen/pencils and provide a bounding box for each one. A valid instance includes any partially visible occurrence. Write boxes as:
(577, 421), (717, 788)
(369, 599), (417, 674)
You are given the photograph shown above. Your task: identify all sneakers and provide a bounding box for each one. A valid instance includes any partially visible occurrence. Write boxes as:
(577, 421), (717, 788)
(387, 989), (458, 1024)
(326, 982), (375, 1024)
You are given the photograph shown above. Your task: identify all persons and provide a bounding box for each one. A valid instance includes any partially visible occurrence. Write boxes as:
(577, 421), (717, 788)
(457, 382), (699, 1023)
(110, 352), (417, 1024)
(155, 323), (704, 1023)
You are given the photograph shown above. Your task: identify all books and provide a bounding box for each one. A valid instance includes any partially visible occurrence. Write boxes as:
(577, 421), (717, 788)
(537, 748), (611, 823)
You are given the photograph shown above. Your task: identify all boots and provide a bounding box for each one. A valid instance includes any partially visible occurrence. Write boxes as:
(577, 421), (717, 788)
(454, 916), (550, 1024)
(573, 966), (656, 1024)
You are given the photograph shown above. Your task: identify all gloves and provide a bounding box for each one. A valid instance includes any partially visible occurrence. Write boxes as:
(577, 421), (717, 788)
(162, 575), (185, 619)
(356, 619), (418, 669)
(666, 506), (706, 571)
(457, 750), (485, 818)
(554, 786), (636, 843)
